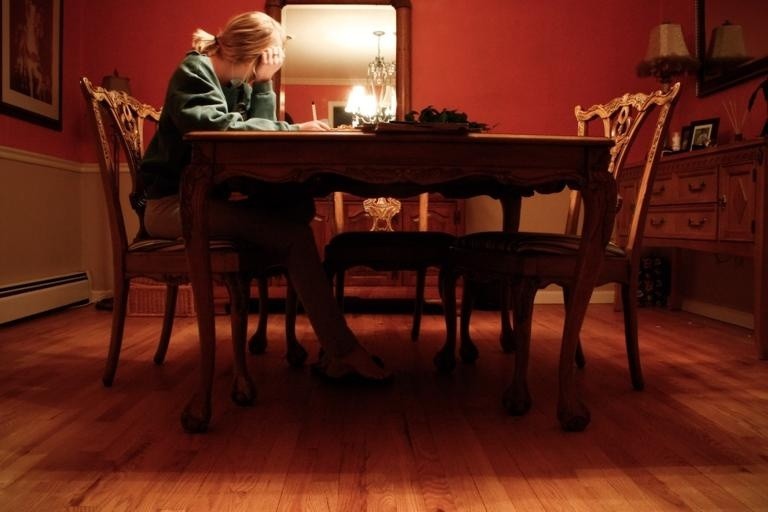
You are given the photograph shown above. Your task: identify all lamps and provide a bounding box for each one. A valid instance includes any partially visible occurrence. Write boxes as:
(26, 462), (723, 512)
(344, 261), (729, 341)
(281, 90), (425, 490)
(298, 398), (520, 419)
(366, 30), (395, 85)
(96, 69), (132, 309)
(704, 18), (754, 75)
(636, 22), (703, 155)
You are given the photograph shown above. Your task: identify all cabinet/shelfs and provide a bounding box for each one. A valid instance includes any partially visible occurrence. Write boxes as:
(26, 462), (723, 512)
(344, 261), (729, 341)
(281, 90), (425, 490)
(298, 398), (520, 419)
(613, 136), (768, 359)
(210, 192), (466, 315)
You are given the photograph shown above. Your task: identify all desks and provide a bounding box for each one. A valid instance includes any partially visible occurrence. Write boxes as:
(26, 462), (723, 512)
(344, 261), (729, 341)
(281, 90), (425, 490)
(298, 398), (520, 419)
(181, 131), (617, 434)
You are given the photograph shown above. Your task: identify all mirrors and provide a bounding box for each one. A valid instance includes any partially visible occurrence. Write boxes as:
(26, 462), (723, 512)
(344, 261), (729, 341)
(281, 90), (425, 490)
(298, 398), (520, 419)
(694, 0), (767, 98)
(266, 1), (413, 130)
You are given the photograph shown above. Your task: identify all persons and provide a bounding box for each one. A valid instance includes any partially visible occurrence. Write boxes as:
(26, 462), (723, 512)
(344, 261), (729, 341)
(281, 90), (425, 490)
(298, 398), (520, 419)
(23, 4), (42, 98)
(133, 11), (395, 392)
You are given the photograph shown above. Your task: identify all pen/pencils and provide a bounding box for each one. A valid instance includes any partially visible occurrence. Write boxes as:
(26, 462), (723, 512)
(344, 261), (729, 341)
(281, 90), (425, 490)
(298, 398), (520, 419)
(311, 102), (317, 121)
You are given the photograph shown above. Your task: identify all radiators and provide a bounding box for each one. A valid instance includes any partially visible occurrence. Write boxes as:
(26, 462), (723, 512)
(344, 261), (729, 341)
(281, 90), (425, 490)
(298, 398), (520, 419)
(0, 267), (91, 325)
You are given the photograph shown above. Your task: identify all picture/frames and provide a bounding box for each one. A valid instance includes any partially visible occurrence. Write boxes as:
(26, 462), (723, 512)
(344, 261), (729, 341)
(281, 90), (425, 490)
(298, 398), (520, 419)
(678, 126), (692, 151)
(0, 0), (63, 131)
(686, 118), (719, 152)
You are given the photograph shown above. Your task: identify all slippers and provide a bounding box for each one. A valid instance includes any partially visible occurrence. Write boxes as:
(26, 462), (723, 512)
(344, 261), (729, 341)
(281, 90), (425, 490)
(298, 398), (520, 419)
(311, 355), (394, 386)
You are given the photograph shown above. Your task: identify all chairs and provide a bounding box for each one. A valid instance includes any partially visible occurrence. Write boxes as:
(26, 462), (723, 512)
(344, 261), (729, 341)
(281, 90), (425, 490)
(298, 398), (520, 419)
(321, 192), (460, 376)
(459, 80), (682, 417)
(80, 75), (308, 407)
